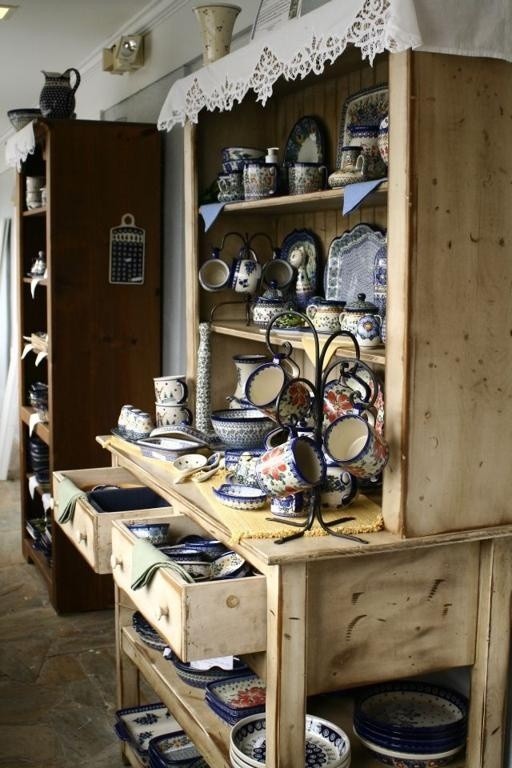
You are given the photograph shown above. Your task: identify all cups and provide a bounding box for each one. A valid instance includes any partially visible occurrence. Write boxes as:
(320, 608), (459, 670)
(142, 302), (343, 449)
(198, 246), (294, 295)
(287, 162), (327, 195)
(253, 423), (328, 518)
(245, 353), (312, 427)
(153, 374), (193, 427)
(242, 162), (278, 202)
(323, 371), (390, 481)
(117, 404), (153, 441)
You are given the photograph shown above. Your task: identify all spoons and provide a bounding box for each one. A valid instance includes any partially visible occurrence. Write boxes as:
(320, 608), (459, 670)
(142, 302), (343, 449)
(173, 452), (220, 484)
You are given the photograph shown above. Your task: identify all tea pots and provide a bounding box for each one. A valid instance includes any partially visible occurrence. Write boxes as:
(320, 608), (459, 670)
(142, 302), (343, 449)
(328, 146), (367, 189)
(317, 454), (360, 510)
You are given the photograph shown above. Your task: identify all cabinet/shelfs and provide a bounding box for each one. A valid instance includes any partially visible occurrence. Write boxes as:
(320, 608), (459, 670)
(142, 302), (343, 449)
(5, 118), (163, 616)
(51, 1), (511, 768)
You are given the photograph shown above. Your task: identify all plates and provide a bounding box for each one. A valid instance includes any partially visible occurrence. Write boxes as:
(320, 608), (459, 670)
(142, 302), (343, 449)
(345, 366), (385, 435)
(337, 81), (389, 152)
(282, 116), (325, 164)
(113, 702), (202, 768)
(352, 679), (469, 768)
(279, 227), (320, 293)
(204, 676), (267, 724)
(323, 223), (387, 309)
(229, 710), (352, 768)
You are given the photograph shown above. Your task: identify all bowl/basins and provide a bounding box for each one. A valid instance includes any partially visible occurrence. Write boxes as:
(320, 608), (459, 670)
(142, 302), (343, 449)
(6, 108), (77, 131)
(216, 145), (264, 203)
(28, 382), (48, 411)
(173, 453), (208, 473)
(132, 610), (170, 652)
(211, 408), (274, 446)
(129, 523), (251, 582)
(175, 650), (249, 689)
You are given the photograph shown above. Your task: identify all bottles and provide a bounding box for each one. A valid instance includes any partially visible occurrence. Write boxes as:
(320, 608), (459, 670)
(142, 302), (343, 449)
(305, 293), (379, 335)
(356, 312), (382, 349)
